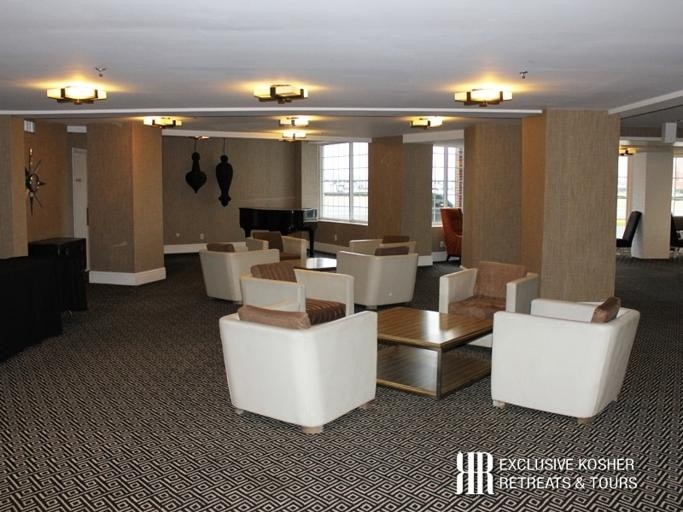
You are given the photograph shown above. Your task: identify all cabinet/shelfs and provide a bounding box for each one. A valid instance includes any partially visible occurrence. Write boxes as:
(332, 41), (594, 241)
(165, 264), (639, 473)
(24, 236), (91, 272)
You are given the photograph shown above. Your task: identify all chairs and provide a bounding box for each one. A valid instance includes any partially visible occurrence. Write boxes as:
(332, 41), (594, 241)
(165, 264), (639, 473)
(194, 205), (683, 438)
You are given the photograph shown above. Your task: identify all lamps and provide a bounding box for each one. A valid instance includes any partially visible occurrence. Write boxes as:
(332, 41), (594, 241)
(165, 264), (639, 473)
(39, 80), (109, 109)
(617, 146), (637, 157)
(405, 116), (446, 132)
(138, 113), (186, 130)
(272, 115), (314, 128)
(279, 129), (310, 142)
(449, 87), (518, 110)
(248, 81), (313, 107)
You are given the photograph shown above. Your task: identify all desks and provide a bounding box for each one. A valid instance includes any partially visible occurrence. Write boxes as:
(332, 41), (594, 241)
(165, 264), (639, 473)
(0, 254), (91, 369)
